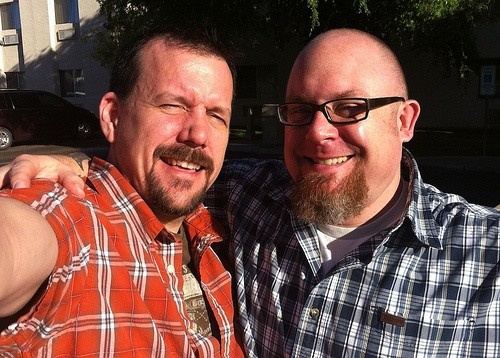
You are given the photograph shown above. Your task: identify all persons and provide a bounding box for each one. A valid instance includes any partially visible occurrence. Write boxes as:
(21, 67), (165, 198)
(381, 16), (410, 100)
(0, 16), (244, 358)
(0, 27), (500, 358)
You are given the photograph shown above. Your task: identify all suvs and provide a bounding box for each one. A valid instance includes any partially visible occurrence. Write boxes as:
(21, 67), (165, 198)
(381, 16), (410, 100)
(0, 88), (100, 151)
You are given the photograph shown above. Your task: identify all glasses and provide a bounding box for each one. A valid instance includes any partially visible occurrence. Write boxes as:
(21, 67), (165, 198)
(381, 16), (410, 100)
(276, 96), (405, 127)
